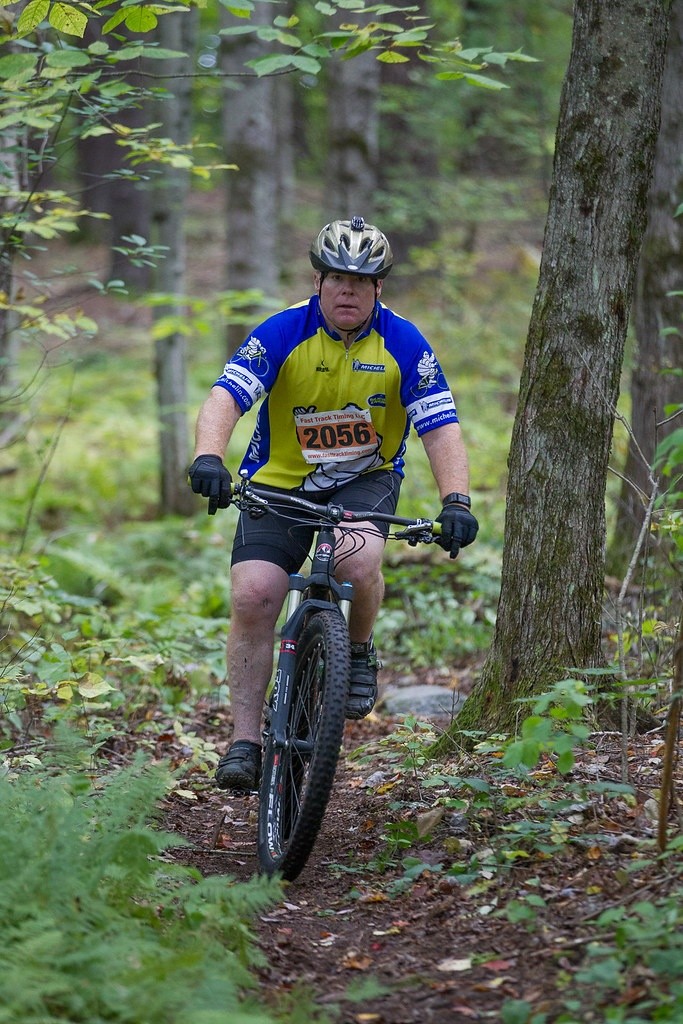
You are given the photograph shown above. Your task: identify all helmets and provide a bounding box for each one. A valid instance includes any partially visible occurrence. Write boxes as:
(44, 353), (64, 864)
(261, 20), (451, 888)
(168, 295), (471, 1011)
(310, 217), (393, 278)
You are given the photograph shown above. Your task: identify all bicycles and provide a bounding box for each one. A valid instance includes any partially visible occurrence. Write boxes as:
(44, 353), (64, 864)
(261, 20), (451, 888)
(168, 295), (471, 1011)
(183, 473), (446, 882)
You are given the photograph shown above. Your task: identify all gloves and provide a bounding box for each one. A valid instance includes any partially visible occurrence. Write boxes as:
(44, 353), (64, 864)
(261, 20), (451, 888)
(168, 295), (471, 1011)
(188, 454), (234, 515)
(435, 505), (478, 558)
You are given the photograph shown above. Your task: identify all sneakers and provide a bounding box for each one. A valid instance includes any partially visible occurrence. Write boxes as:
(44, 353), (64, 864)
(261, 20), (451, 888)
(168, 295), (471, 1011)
(346, 633), (382, 720)
(214, 740), (266, 789)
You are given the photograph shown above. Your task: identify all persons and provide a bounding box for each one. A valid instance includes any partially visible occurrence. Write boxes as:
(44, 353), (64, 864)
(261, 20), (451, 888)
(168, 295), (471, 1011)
(181, 216), (479, 791)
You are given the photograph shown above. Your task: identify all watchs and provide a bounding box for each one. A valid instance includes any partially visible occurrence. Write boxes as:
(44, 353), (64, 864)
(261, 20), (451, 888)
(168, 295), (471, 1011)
(440, 491), (471, 507)
